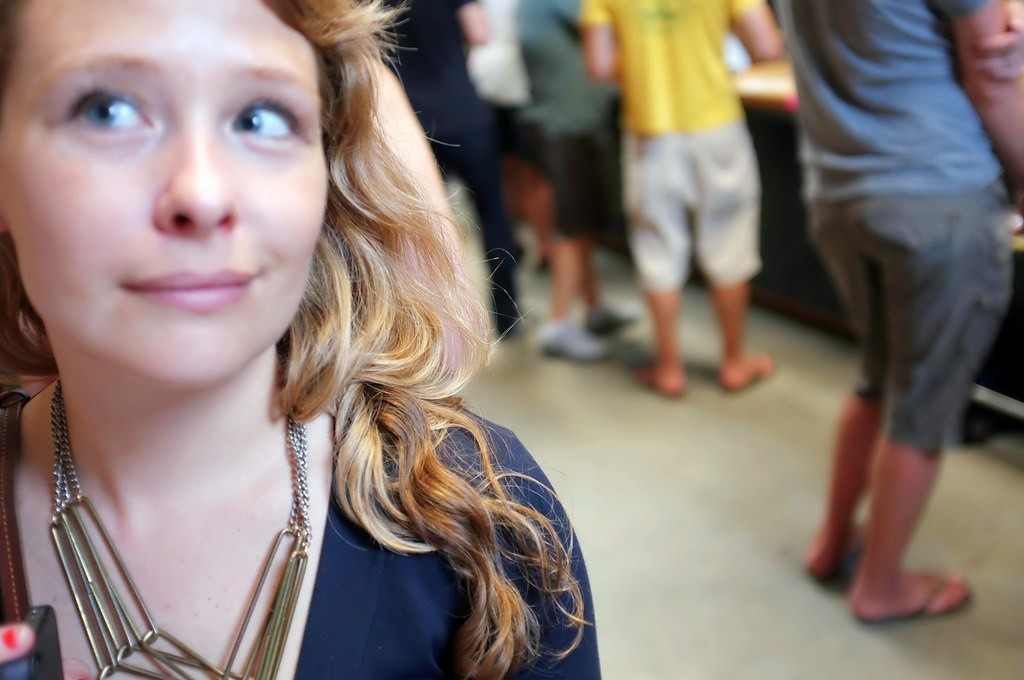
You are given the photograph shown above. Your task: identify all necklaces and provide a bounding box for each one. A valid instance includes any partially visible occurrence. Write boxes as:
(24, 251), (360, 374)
(47, 377), (312, 680)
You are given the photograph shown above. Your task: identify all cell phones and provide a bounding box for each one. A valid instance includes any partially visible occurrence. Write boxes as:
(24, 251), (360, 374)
(0, 605), (64, 680)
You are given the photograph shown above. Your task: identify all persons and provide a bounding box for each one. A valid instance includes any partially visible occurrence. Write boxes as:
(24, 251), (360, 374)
(0, 0), (599, 680)
(367, 0), (628, 381)
(578, 0), (785, 399)
(770, 0), (1024, 622)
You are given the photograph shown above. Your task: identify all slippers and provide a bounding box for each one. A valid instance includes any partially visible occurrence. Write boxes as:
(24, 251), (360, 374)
(807, 535), (862, 583)
(633, 363), (686, 397)
(853, 574), (968, 625)
(724, 349), (775, 391)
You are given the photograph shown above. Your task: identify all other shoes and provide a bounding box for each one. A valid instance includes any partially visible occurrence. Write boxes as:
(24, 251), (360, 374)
(541, 320), (613, 361)
(584, 296), (643, 334)
